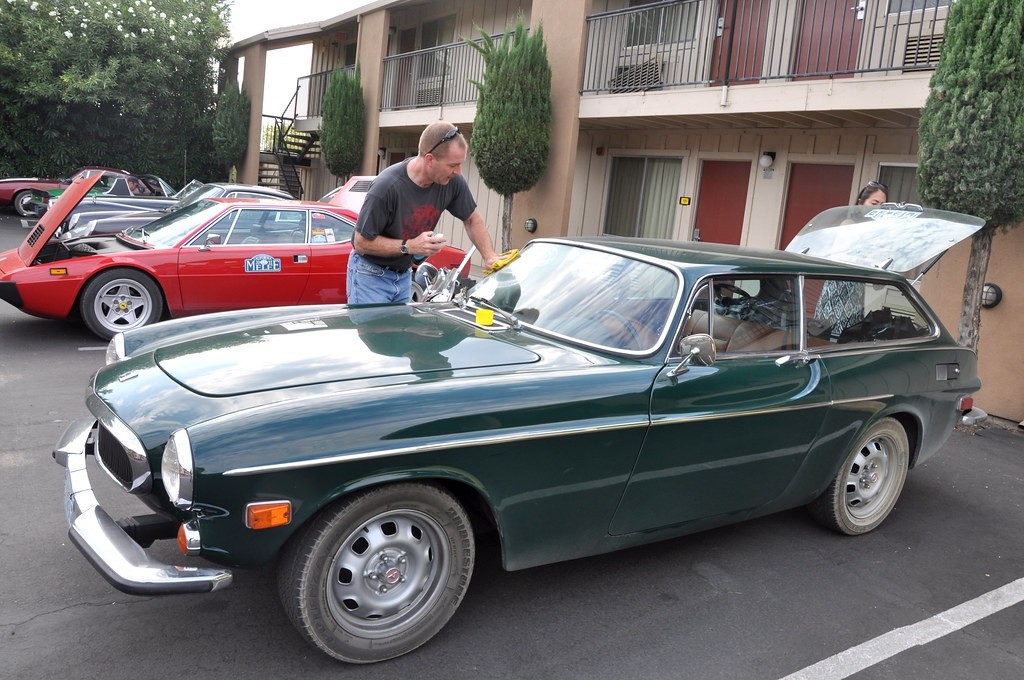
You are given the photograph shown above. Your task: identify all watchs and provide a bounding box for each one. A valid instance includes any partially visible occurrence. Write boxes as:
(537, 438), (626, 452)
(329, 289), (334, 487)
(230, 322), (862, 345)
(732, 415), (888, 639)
(400, 239), (409, 256)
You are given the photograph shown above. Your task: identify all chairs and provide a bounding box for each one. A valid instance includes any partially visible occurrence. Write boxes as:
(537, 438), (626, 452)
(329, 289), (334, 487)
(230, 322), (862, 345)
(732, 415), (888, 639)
(606, 317), (660, 351)
(242, 224), (265, 244)
(291, 220), (306, 243)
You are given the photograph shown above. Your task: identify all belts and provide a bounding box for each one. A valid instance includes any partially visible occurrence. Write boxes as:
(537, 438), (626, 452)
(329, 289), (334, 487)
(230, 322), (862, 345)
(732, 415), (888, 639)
(379, 265), (408, 273)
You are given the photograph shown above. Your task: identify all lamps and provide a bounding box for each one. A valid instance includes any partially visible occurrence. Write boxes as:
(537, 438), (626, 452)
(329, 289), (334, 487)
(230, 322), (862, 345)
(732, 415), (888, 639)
(524, 217), (537, 233)
(378, 146), (386, 160)
(981, 283), (1003, 308)
(760, 151), (776, 171)
(389, 25), (397, 35)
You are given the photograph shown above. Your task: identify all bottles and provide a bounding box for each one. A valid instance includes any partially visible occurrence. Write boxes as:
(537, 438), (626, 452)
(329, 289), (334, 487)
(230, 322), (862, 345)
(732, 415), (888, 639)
(411, 234), (444, 265)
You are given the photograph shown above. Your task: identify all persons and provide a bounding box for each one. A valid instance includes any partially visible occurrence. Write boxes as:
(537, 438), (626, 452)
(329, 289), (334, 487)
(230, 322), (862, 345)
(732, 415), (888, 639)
(814, 183), (888, 334)
(127, 176), (140, 193)
(346, 122), (510, 303)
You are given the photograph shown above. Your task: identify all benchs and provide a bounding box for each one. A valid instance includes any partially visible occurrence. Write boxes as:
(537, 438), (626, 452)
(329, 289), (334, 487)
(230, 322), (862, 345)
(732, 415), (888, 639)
(681, 308), (834, 355)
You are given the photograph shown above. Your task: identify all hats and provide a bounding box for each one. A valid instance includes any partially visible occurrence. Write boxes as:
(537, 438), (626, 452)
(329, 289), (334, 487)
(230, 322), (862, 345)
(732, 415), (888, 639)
(127, 176), (139, 185)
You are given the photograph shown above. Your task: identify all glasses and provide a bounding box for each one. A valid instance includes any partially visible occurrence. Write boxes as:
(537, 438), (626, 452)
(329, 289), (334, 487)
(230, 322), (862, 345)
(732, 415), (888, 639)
(869, 180), (889, 191)
(424, 126), (460, 156)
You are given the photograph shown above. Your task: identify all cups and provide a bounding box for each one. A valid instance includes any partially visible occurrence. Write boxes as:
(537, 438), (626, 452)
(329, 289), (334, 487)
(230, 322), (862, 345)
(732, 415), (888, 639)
(475, 308), (494, 326)
